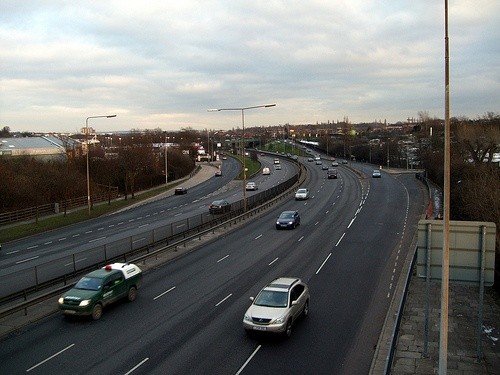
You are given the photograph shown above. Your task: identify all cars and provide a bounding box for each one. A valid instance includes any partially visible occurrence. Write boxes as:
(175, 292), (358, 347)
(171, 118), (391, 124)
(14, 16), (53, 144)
(329, 158), (348, 166)
(215, 171), (223, 177)
(276, 152), (299, 160)
(276, 164), (283, 170)
(209, 202), (231, 213)
(262, 167), (272, 175)
(173, 187), (189, 196)
(302, 146), (328, 170)
(222, 154), (228, 160)
(276, 211), (301, 229)
(373, 170), (382, 178)
(294, 188), (311, 200)
(245, 182), (259, 191)
(273, 159), (281, 164)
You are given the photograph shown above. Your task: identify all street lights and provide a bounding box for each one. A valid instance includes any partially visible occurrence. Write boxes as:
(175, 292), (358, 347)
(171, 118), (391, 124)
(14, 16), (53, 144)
(118, 137), (122, 149)
(131, 135), (134, 149)
(160, 136), (183, 148)
(87, 115), (117, 216)
(207, 104), (279, 212)
(104, 135), (113, 147)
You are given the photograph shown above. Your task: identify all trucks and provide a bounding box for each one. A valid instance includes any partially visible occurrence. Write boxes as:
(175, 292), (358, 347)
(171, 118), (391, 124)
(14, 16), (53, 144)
(59, 263), (143, 320)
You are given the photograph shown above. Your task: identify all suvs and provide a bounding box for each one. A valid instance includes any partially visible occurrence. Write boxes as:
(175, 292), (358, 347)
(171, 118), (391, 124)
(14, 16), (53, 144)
(242, 277), (310, 336)
(326, 168), (337, 178)
